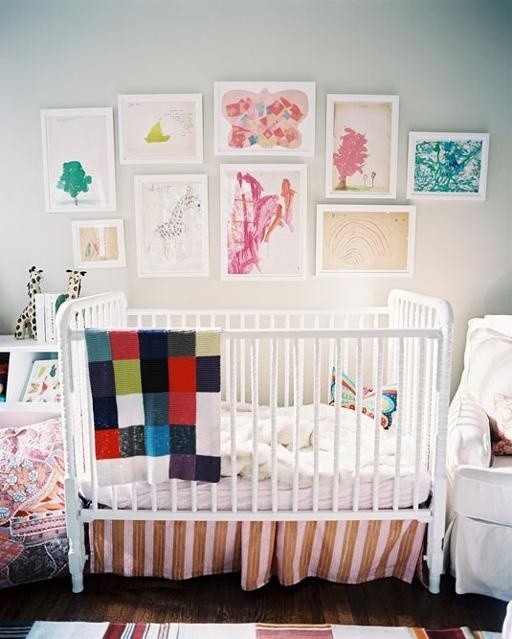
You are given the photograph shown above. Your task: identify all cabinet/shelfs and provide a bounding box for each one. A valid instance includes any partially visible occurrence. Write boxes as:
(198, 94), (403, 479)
(0, 335), (59, 403)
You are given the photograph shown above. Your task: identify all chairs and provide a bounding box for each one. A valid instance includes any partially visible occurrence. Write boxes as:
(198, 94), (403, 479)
(446, 315), (512, 602)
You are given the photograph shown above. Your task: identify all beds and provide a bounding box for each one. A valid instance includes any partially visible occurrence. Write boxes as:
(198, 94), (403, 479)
(55, 289), (454, 594)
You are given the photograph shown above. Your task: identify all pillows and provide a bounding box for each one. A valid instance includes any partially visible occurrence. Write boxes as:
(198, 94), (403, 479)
(470, 392), (511, 455)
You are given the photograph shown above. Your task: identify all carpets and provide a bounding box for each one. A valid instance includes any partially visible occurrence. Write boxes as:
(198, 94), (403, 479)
(0, 621), (503, 639)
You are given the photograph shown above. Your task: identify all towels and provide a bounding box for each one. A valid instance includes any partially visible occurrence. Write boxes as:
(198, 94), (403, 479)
(84, 327), (223, 485)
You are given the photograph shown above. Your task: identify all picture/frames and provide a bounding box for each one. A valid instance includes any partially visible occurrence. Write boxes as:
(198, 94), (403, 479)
(118, 94), (203, 164)
(219, 163), (308, 282)
(134, 173), (210, 278)
(406, 131), (490, 202)
(41, 108), (117, 211)
(213, 81), (316, 155)
(315, 204), (416, 278)
(72, 219), (127, 269)
(325, 93), (400, 199)
